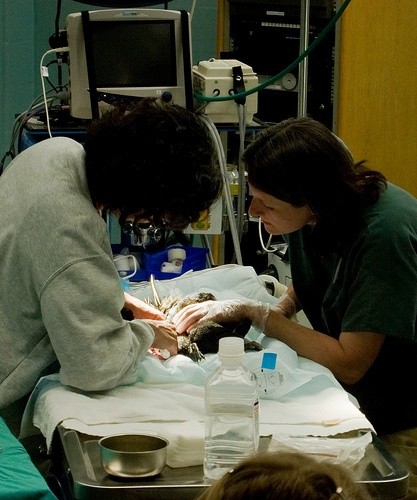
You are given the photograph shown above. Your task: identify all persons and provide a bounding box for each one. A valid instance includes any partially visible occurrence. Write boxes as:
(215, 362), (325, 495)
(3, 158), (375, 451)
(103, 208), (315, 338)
(194, 449), (375, 500)
(0, 92), (223, 420)
(172, 116), (416, 499)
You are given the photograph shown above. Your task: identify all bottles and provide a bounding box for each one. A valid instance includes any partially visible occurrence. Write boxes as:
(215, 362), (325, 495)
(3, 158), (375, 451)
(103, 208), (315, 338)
(204, 337), (261, 480)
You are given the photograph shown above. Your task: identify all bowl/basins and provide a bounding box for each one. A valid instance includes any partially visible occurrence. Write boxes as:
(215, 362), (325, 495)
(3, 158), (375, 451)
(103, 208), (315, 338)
(99, 433), (169, 477)
